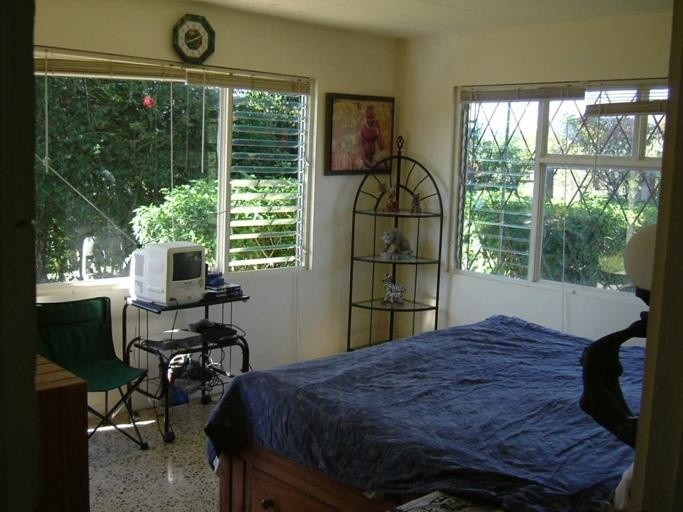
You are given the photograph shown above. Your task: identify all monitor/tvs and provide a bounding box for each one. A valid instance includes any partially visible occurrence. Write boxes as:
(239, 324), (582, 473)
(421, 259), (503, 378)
(130, 239), (205, 306)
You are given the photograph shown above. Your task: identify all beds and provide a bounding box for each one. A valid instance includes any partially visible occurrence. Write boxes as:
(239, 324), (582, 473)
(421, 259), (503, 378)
(216, 315), (647, 512)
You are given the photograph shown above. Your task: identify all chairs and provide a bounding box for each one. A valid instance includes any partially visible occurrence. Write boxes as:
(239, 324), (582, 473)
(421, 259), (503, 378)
(35, 297), (150, 452)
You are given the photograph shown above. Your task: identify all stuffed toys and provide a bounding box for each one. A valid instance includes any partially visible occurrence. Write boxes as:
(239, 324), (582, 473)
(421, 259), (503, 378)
(380, 273), (405, 305)
(382, 185), (399, 213)
(409, 193), (421, 214)
(380, 227), (413, 260)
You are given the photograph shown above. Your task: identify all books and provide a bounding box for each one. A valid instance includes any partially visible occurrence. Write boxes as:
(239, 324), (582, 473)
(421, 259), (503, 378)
(205, 282), (240, 294)
(206, 290), (242, 299)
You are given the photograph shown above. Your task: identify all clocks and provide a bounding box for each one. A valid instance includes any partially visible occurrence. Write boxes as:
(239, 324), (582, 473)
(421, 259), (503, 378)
(173, 12), (215, 68)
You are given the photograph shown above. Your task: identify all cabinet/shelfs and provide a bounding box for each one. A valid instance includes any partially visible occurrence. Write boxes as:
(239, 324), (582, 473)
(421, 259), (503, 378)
(34, 351), (89, 512)
(117, 292), (251, 441)
(347, 133), (444, 350)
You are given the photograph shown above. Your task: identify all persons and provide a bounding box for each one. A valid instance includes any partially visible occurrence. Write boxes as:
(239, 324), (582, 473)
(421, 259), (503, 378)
(357, 105), (390, 169)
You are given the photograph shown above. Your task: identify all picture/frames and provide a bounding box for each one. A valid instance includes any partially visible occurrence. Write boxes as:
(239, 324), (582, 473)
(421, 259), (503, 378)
(322, 90), (395, 177)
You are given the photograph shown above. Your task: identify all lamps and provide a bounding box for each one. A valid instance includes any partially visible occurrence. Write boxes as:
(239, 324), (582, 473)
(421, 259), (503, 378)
(623, 225), (657, 307)
